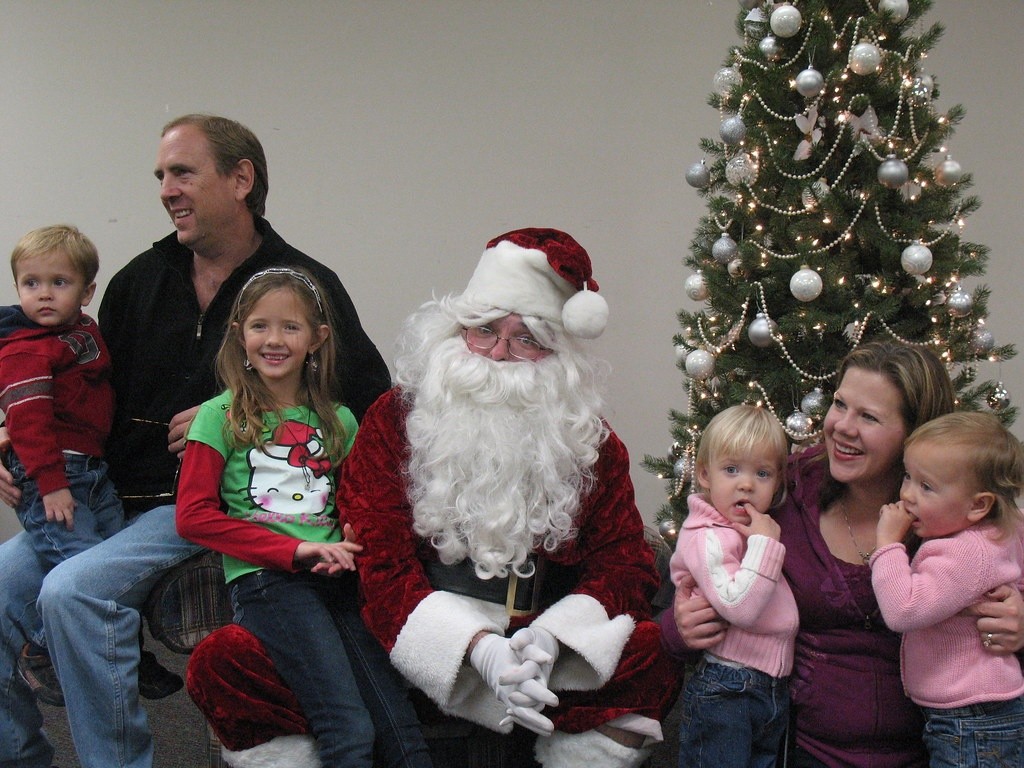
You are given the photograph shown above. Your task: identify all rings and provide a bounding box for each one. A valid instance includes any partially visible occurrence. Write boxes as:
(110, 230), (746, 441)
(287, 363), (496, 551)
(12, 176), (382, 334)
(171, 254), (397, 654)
(984, 634), (992, 646)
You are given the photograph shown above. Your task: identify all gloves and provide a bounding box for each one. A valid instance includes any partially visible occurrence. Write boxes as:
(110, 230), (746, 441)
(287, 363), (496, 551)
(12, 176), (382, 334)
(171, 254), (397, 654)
(472, 628), (560, 738)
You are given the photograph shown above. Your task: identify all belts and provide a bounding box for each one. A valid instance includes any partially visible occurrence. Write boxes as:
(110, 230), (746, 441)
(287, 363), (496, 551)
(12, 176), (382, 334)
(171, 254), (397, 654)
(415, 539), (570, 616)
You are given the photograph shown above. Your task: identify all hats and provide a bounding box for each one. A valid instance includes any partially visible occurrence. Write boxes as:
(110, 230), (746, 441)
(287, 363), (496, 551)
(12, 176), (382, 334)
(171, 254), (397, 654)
(463, 227), (610, 339)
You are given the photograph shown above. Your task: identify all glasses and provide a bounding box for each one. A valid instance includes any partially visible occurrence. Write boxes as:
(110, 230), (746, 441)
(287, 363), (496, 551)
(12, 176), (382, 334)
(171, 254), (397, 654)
(119, 418), (183, 500)
(462, 326), (549, 359)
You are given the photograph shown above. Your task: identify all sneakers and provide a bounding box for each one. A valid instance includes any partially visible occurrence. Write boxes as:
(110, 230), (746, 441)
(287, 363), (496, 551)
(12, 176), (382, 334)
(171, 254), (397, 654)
(17, 644), (66, 707)
(136, 654), (185, 700)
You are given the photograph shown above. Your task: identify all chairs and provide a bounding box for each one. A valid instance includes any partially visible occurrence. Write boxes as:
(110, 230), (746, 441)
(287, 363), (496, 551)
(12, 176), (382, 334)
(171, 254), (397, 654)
(147, 527), (674, 768)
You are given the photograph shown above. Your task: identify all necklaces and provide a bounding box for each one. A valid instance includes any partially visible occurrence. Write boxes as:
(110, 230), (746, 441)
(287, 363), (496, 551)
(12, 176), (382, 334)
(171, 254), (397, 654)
(841, 504), (876, 565)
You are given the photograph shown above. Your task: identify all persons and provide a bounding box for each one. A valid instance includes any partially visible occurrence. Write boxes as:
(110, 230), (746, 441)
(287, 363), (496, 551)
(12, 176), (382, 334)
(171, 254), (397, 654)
(175, 264), (433, 768)
(0, 113), (391, 768)
(870, 412), (1024, 768)
(669, 405), (800, 768)
(187, 228), (684, 768)
(0, 224), (184, 706)
(660, 342), (1024, 768)
